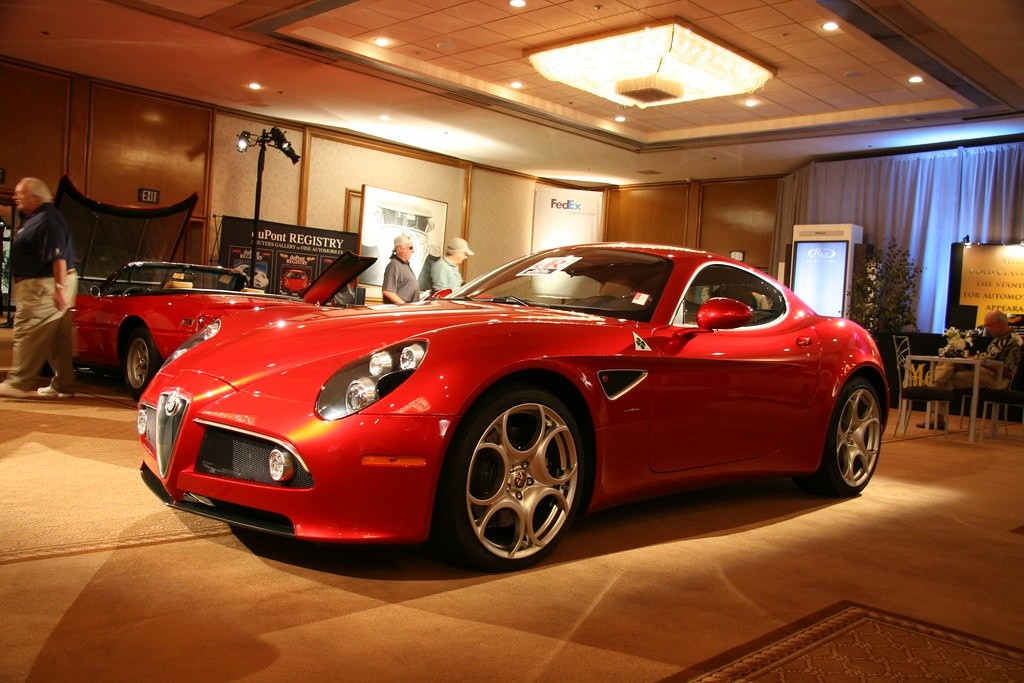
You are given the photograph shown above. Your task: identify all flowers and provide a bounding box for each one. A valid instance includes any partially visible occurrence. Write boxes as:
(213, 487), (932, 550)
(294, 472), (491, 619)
(939, 327), (972, 346)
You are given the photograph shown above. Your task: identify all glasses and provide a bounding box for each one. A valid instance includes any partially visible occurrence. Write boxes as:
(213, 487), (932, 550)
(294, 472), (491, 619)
(983, 319), (1002, 329)
(410, 247), (413, 250)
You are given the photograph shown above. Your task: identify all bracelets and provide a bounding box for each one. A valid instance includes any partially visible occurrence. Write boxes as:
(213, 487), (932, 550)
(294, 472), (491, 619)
(55, 281), (64, 289)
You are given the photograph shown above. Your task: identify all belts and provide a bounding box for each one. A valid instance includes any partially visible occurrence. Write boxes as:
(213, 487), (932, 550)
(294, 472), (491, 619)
(14, 270), (77, 284)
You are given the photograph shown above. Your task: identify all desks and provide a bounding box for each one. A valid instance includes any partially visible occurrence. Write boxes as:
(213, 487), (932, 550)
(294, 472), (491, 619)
(901, 355), (1003, 444)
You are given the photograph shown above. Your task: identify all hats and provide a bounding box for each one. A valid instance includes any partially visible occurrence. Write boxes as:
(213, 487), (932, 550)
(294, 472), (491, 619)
(394, 235), (413, 246)
(447, 238), (474, 256)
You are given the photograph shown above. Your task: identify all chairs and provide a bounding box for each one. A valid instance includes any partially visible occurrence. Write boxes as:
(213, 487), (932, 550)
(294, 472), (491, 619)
(893, 335), (951, 442)
(960, 375), (1013, 442)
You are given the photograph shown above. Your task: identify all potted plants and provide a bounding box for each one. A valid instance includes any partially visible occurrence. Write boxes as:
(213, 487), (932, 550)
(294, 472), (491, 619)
(845, 236), (923, 406)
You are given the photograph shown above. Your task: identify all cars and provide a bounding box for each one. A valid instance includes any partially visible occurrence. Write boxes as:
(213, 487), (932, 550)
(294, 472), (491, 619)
(70, 251), (379, 400)
(137, 240), (891, 575)
(281, 269), (310, 292)
(234, 264), (269, 289)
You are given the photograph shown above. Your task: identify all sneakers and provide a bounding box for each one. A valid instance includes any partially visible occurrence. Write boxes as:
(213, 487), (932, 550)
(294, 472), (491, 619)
(0, 381), (38, 398)
(37, 386), (74, 398)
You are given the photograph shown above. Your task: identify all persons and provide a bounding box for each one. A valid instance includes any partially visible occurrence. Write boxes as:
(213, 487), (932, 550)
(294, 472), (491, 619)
(0, 177), (79, 398)
(915, 310), (1023, 430)
(430, 238), (475, 293)
(382, 234), (420, 304)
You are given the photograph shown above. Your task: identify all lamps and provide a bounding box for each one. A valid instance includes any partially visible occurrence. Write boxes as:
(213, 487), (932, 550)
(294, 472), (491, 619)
(266, 127), (292, 150)
(282, 147), (302, 166)
(236, 131), (259, 153)
(522, 17), (778, 110)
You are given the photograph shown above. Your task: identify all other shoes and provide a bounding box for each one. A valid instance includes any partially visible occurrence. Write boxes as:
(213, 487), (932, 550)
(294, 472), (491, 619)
(917, 421), (945, 430)
(903, 386), (948, 400)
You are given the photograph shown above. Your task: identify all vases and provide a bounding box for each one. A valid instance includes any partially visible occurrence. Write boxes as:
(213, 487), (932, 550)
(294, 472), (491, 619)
(950, 338), (966, 350)
